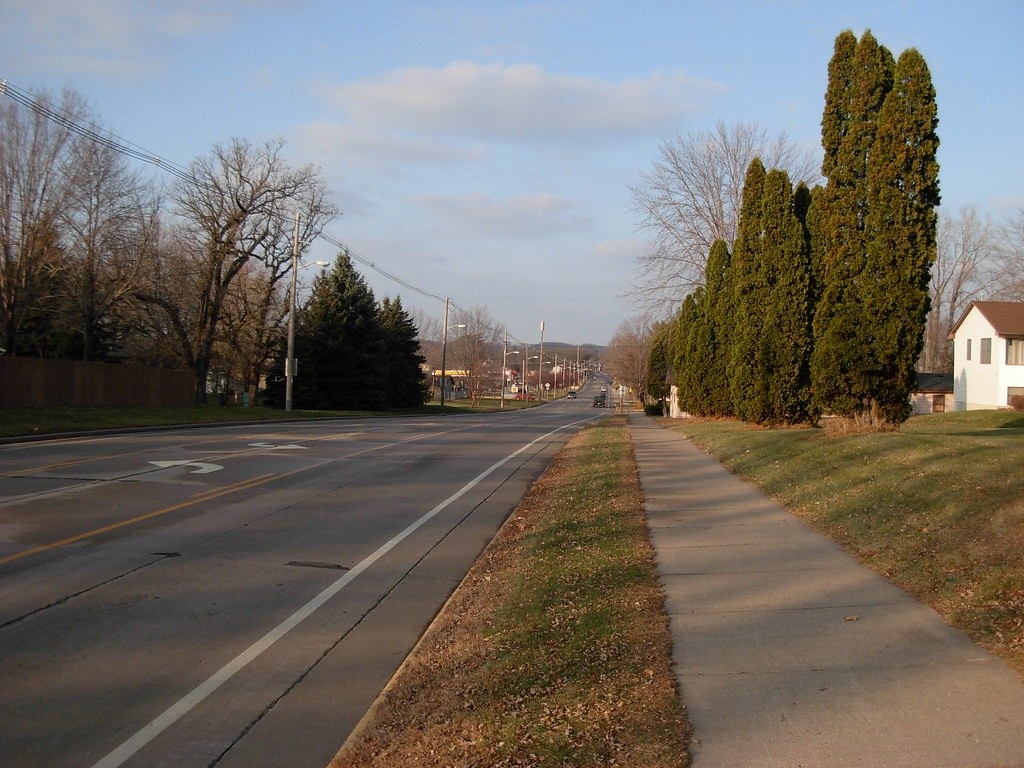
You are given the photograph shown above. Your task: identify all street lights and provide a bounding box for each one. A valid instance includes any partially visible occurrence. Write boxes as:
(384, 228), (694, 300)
(561, 367), (576, 395)
(526, 356), (539, 405)
(501, 351), (519, 409)
(441, 324), (466, 413)
(538, 362), (551, 402)
(555, 365), (564, 399)
(285, 259), (330, 413)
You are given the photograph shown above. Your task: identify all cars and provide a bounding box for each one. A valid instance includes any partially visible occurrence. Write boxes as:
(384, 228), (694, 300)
(591, 374), (613, 397)
(567, 392), (576, 399)
(514, 392), (536, 401)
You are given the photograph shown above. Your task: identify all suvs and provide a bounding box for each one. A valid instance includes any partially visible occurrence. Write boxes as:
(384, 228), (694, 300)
(594, 396), (606, 407)
